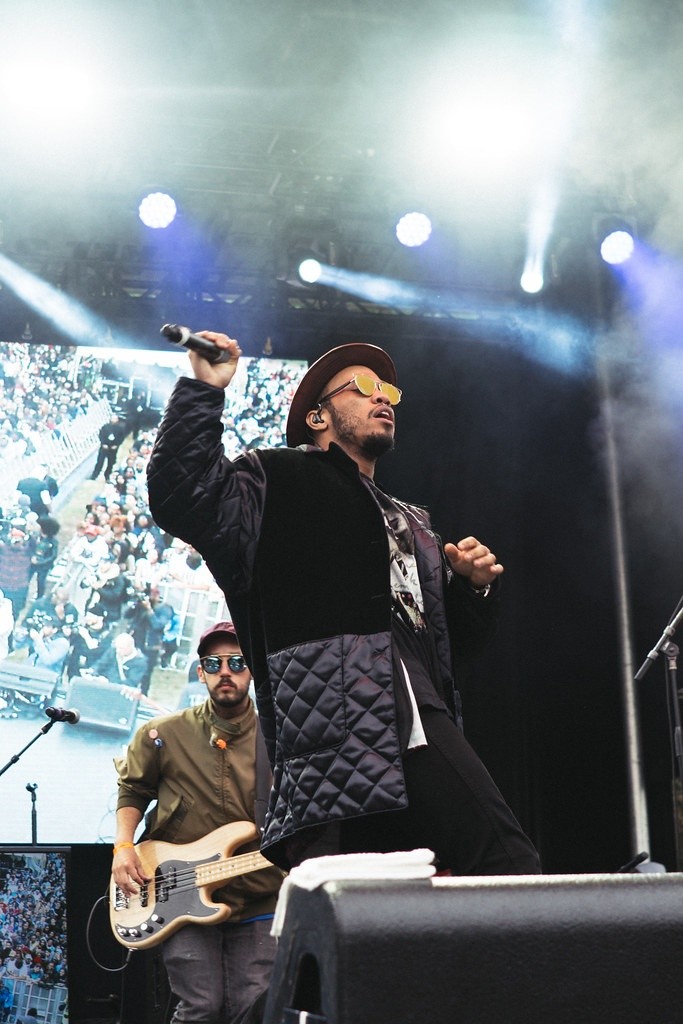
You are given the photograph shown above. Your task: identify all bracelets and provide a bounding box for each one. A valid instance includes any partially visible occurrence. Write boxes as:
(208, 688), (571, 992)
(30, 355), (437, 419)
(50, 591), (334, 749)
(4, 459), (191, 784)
(112, 841), (133, 854)
(466, 584), (490, 596)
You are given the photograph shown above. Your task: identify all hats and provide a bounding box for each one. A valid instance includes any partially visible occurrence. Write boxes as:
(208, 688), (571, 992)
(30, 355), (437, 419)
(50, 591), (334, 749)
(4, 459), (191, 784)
(197, 621), (238, 656)
(286, 343), (397, 448)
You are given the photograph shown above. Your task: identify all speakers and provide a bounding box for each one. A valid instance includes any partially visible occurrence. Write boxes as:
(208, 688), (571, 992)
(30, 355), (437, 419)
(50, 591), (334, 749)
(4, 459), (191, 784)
(263, 871), (683, 1024)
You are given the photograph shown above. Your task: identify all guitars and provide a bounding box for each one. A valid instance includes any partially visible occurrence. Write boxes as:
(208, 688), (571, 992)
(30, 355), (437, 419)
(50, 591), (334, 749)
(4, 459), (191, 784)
(108, 820), (279, 952)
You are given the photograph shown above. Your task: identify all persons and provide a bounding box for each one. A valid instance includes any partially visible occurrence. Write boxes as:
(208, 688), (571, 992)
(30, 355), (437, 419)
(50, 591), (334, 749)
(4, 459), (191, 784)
(146, 332), (539, 885)
(110, 620), (288, 1024)
(0, 343), (308, 687)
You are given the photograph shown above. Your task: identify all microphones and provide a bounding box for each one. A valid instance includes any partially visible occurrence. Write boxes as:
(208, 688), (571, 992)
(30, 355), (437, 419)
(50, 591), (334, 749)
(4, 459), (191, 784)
(159, 321), (235, 365)
(45, 705), (83, 725)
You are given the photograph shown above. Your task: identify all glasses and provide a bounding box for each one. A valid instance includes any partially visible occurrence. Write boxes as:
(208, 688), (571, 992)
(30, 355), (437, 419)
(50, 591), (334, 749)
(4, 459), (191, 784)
(317, 374), (403, 405)
(199, 654), (248, 675)
